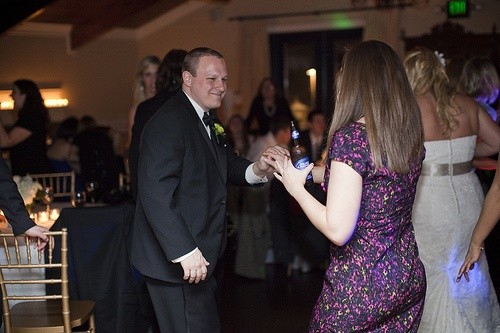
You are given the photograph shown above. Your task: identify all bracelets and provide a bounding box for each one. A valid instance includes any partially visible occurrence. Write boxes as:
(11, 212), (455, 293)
(471, 240), (485, 251)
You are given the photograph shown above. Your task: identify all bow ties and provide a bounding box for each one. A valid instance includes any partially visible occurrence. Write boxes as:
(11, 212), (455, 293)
(203, 111), (217, 128)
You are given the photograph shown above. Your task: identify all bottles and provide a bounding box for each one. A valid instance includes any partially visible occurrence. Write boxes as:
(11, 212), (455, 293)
(287, 120), (315, 188)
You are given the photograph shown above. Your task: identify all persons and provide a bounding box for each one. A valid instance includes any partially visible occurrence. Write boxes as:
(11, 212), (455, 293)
(445, 52), (499, 120)
(128, 50), (189, 199)
(227, 75), (327, 167)
(0, 153), (56, 255)
(122, 45), (291, 333)
(392, 44), (500, 333)
(0, 78), (131, 205)
(264, 38), (428, 333)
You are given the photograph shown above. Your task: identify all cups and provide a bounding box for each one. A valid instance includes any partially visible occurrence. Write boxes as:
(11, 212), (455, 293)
(74, 192), (86, 209)
(85, 179), (99, 204)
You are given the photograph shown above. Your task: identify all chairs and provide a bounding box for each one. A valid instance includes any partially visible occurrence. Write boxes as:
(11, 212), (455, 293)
(0, 227), (96, 333)
(27, 172), (75, 202)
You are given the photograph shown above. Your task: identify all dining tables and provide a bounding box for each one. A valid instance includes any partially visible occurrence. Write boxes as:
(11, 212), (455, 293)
(1, 199), (140, 333)
